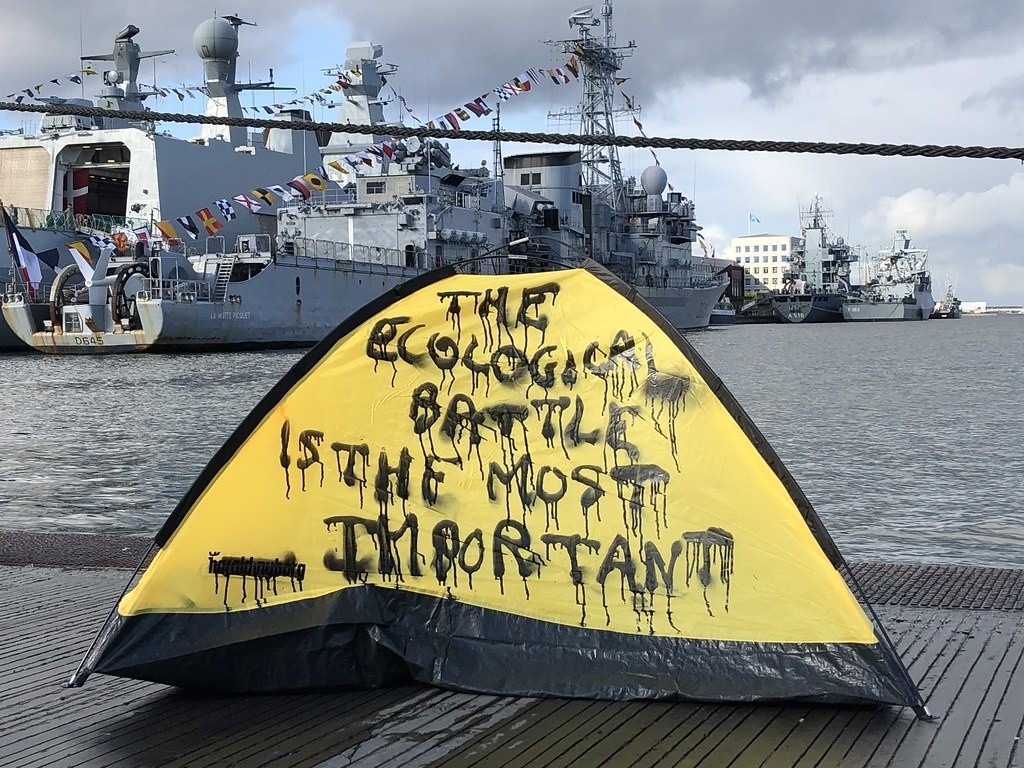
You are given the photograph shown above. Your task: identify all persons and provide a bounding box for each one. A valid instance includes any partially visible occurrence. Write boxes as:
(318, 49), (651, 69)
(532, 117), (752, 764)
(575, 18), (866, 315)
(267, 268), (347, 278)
(62, 203), (73, 229)
(645, 269), (669, 288)
(9, 204), (18, 224)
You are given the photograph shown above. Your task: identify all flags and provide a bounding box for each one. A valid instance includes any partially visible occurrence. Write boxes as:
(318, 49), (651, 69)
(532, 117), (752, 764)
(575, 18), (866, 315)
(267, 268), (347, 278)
(266, 184), (294, 203)
(379, 44), (708, 258)
(7, 62), (363, 115)
(316, 165), (330, 183)
(232, 193), (262, 214)
(195, 208), (223, 236)
(329, 145), (384, 174)
(285, 180), (313, 200)
(1, 206), (43, 303)
(302, 172), (326, 192)
(249, 187), (276, 207)
(155, 221), (179, 246)
(750, 213), (760, 223)
(211, 198), (237, 223)
(65, 225), (152, 265)
(176, 215), (199, 240)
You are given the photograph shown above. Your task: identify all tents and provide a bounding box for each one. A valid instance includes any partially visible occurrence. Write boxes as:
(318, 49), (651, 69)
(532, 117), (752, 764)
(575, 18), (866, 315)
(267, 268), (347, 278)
(63, 234), (941, 722)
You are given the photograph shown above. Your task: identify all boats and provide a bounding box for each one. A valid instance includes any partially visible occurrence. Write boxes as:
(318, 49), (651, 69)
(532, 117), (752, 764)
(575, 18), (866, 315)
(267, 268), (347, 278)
(840, 228), (936, 322)
(767, 193), (865, 325)
(0, 0), (736, 355)
(929, 275), (963, 319)
